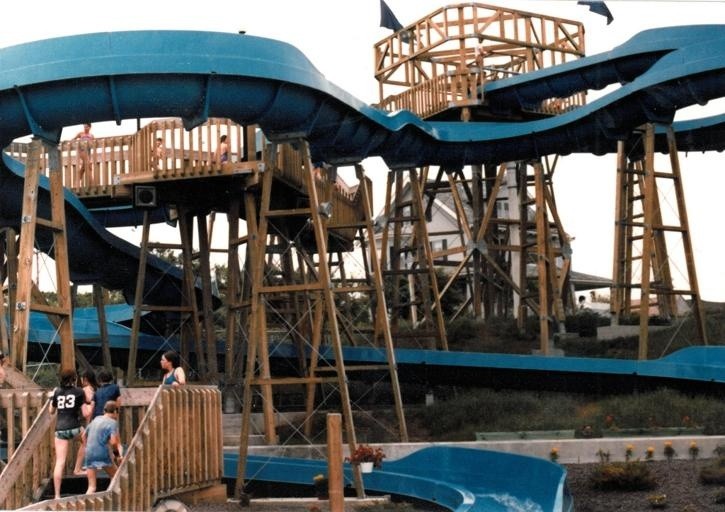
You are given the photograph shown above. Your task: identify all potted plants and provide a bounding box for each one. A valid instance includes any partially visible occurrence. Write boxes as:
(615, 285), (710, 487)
(343, 446), (387, 474)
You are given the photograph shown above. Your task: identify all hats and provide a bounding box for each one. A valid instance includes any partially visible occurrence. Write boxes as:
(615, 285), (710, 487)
(103, 401), (120, 413)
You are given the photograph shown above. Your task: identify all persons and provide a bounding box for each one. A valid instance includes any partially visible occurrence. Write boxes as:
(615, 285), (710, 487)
(80, 371), (99, 424)
(150, 138), (162, 172)
(70, 123), (96, 187)
(160, 351), (186, 386)
(48, 368), (87, 500)
(90, 370), (122, 417)
(578, 295), (589, 308)
(79, 400), (123, 497)
(213, 135), (230, 163)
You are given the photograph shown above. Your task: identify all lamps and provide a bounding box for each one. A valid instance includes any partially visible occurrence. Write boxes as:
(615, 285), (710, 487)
(319, 200), (335, 218)
(161, 204), (179, 228)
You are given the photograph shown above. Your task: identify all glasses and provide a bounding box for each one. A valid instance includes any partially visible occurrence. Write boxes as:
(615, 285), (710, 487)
(103, 408), (113, 413)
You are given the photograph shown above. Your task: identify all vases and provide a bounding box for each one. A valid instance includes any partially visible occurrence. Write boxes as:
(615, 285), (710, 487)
(317, 479), (329, 499)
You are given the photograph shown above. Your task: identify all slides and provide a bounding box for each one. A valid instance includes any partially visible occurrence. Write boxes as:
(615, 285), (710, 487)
(1, 26), (725, 507)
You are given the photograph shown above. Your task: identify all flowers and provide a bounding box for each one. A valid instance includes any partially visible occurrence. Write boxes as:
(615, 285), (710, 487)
(313, 474), (324, 480)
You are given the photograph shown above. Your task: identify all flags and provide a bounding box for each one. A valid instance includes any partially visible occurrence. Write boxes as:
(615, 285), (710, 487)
(577, 2), (614, 26)
(380, 0), (409, 45)
